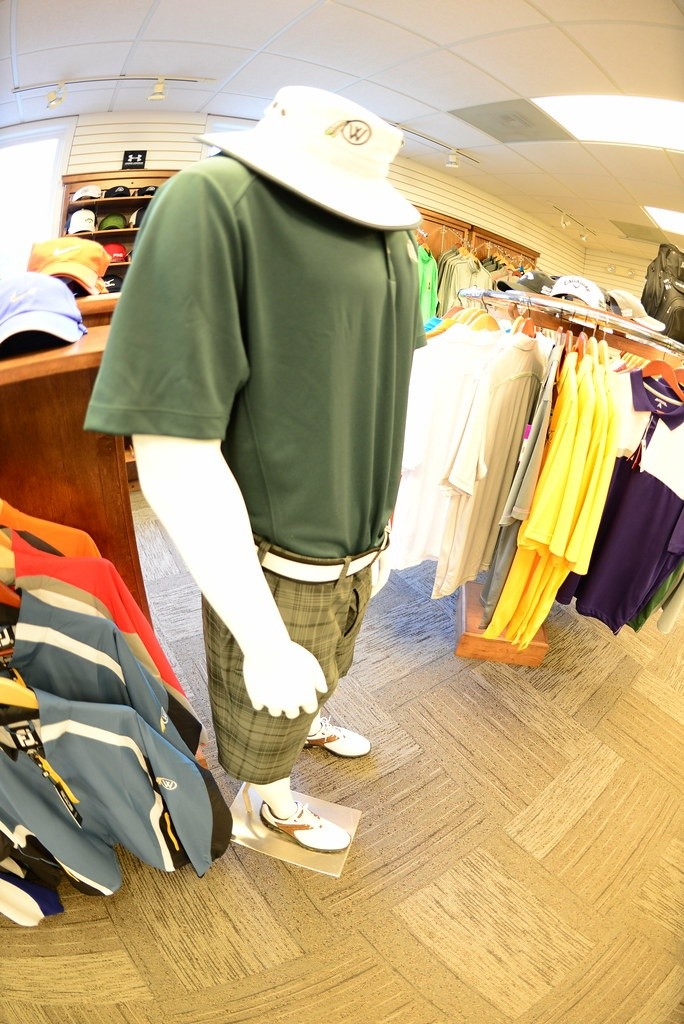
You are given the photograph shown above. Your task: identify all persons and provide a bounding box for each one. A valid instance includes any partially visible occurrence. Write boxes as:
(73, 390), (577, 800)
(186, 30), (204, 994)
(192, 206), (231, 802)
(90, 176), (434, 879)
(82, 84), (426, 854)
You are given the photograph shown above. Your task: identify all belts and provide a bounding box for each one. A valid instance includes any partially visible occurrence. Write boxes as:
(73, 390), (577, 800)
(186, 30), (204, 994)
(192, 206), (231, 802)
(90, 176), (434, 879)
(255, 519), (394, 582)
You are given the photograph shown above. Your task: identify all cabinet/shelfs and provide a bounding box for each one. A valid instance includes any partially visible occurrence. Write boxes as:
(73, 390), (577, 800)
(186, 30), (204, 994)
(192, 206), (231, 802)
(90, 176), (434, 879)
(66, 195), (156, 267)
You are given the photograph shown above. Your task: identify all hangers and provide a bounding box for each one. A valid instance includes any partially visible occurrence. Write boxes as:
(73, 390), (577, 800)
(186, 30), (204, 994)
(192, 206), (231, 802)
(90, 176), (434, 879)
(0, 495), (62, 718)
(408, 224), (543, 278)
(431, 285), (680, 386)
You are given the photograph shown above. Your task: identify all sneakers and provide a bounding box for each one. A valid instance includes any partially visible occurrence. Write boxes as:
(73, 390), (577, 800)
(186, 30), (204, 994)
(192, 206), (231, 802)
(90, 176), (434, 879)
(303, 717), (371, 759)
(260, 796), (352, 854)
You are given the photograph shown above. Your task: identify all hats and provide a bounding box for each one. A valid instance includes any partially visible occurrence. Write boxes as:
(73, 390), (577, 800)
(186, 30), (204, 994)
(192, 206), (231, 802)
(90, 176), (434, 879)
(498, 271), (667, 332)
(194, 87), (422, 232)
(0, 186), (160, 353)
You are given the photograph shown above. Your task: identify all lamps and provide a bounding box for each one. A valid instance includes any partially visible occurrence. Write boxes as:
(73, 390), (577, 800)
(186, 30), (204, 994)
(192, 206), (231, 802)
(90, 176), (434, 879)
(561, 211), (571, 230)
(48, 84), (71, 107)
(445, 147), (461, 168)
(576, 227), (587, 243)
(148, 76), (168, 102)
